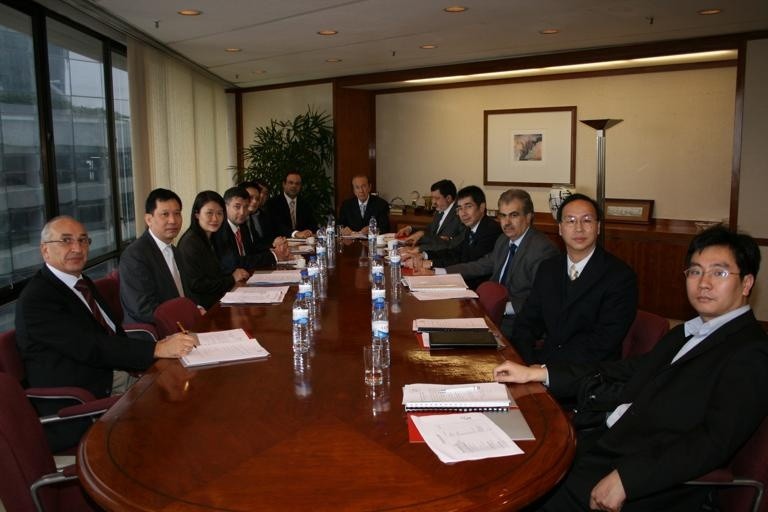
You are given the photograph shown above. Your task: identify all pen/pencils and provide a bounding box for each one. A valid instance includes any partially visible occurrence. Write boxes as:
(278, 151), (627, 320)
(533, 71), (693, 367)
(176, 321), (197, 349)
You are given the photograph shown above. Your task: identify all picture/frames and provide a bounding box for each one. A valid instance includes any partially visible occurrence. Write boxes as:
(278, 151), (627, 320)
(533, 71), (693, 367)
(482, 105), (577, 190)
(604, 199), (655, 226)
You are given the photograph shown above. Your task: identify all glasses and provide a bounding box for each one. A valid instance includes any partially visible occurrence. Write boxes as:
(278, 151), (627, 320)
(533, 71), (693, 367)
(42, 237), (92, 246)
(682, 265), (745, 280)
(561, 215), (598, 226)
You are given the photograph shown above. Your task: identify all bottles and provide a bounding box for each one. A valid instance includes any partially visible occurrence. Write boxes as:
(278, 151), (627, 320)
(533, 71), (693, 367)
(372, 390), (391, 434)
(369, 252), (386, 299)
(390, 246), (401, 285)
(326, 214), (336, 248)
(294, 353), (313, 400)
(308, 257), (321, 299)
(316, 238), (327, 272)
(298, 270), (314, 325)
(371, 302), (391, 369)
(292, 293), (310, 353)
(368, 215), (377, 257)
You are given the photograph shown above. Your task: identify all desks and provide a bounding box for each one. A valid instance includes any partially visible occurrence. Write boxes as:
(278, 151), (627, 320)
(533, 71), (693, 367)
(77, 232), (581, 511)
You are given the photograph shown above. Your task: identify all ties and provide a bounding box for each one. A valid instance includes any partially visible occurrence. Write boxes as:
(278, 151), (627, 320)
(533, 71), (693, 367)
(361, 204), (365, 218)
(469, 230), (472, 246)
(290, 200), (297, 228)
(501, 243), (517, 283)
(249, 214), (260, 245)
(236, 227), (245, 257)
(567, 264), (576, 281)
(76, 280), (117, 344)
(166, 245), (186, 297)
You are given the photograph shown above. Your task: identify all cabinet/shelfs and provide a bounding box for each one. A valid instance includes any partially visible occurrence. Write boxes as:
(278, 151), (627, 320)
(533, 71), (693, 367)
(603, 230), (700, 320)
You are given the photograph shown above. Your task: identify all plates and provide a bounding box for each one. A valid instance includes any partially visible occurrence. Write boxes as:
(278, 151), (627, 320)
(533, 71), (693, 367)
(377, 244), (386, 248)
(305, 242), (316, 245)
(292, 265), (306, 269)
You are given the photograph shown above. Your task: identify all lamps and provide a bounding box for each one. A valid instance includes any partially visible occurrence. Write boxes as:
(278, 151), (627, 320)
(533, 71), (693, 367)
(578, 117), (625, 247)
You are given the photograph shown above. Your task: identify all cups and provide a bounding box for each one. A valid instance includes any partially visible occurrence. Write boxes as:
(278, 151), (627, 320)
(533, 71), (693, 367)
(337, 225), (343, 238)
(377, 235), (384, 244)
(306, 237), (315, 243)
(296, 259), (306, 267)
(363, 345), (384, 386)
(413, 255), (423, 274)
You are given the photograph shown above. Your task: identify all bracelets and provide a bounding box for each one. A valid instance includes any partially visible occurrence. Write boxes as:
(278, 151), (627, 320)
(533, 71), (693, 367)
(196, 304), (204, 308)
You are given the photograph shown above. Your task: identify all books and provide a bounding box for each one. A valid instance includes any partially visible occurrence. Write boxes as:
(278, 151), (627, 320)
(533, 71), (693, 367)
(403, 381), (537, 443)
(411, 317), (497, 351)
(174, 328), (270, 367)
(221, 285), (288, 303)
(402, 274), (478, 302)
(250, 265), (319, 285)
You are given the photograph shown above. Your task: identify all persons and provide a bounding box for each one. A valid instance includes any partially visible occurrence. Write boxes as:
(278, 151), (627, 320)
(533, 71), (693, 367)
(490, 221), (768, 511)
(512, 195), (638, 371)
(119, 187), (207, 323)
(177, 191), (247, 303)
(15, 214), (199, 453)
(216, 171), (563, 334)
